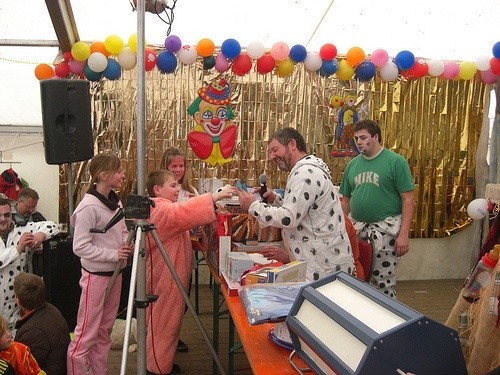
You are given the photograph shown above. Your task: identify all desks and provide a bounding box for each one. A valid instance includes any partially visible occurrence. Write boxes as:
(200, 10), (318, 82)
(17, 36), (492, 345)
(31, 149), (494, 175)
(194, 249), (317, 375)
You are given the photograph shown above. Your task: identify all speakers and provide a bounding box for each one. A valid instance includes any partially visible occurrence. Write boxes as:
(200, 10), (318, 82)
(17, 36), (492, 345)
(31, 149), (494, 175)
(40, 79), (94, 165)
(32, 238), (82, 333)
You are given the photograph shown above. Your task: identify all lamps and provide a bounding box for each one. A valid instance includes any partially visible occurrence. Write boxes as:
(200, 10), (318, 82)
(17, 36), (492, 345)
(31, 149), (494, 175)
(129, 0), (178, 36)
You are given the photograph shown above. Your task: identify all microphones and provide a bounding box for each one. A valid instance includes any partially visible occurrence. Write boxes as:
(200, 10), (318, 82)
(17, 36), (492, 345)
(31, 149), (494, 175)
(259, 174), (267, 206)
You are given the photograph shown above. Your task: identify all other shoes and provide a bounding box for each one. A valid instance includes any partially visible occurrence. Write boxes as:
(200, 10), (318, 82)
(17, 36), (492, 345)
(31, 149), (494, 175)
(177, 338), (189, 352)
(147, 363), (180, 375)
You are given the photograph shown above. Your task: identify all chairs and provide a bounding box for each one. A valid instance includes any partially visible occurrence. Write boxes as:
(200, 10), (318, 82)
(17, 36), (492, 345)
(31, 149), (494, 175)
(358, 236), (375, 285)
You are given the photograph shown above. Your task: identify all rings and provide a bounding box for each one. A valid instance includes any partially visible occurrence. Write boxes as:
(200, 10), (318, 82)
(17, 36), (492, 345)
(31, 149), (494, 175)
(34, 241), (38, 245)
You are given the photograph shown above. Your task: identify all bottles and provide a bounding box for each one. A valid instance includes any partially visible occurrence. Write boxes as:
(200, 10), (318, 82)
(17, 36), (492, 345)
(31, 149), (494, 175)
(457, 313), (474, 363)
(489, 270), (500, 315)
(461, 244), (500, 304)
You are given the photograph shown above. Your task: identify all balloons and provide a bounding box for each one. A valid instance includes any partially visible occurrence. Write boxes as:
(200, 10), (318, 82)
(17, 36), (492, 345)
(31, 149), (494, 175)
(466, 198), (489, 221)
(34, 32), (500, 86)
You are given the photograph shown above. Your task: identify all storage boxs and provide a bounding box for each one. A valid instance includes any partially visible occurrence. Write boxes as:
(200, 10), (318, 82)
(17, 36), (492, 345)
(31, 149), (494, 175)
(206, 203), (232, 277)
(246, 267), (277, 286)
(268, 259), (308, 283)
(226, 252), (254, 281)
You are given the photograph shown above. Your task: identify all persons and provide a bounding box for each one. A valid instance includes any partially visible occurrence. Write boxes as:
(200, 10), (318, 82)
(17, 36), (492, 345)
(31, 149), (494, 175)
(0, 197), (62, 341)
(11, 271), (71, 375)
(233, 127), (355, 283)
(261, 212), (366, 280)
(159, 146), (204, 354)
(11, 188), (53, 275)
(0, 315), (47, 375)
(338, 119), (416, 302)
(65, 151), (135, 375)
(142, 169), (236, 375)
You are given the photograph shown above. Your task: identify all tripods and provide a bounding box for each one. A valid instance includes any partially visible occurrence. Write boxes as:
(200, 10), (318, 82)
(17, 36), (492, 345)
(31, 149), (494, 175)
(102, 217), (228, 375)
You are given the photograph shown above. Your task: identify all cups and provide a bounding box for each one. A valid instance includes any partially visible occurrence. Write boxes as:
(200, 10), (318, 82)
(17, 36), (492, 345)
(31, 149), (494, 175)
(244, 275), (259, 286)
